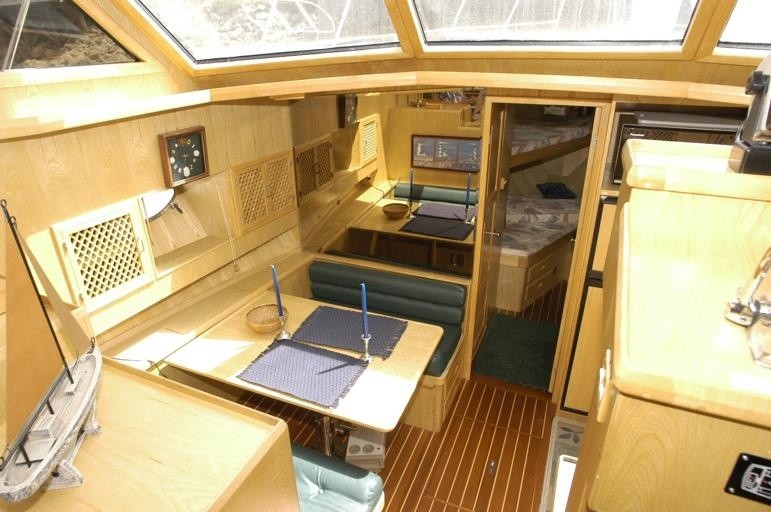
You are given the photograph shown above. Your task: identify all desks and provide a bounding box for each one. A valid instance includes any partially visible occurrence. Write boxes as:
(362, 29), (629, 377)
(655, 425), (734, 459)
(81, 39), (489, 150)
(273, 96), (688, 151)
(350, 198), (476, 277)
(164, 291), (445, 458)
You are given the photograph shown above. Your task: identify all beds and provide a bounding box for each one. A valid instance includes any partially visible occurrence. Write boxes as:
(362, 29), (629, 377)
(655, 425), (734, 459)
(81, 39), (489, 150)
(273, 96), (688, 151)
(494, 121), (593, 318)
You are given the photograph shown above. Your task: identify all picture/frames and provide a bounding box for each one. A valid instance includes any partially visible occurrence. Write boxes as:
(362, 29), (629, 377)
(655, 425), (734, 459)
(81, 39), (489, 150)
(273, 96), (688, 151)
(411, 134), (481, 173)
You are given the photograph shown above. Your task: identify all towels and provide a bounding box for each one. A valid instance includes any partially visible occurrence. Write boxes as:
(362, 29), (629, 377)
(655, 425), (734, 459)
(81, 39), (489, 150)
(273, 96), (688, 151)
(235, 305), (408, 408)
(398, 201), (477, 242)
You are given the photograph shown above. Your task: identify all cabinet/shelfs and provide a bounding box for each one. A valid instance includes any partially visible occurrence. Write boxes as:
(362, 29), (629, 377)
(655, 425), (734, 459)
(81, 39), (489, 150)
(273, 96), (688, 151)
(544, 138), (771, 512)
(0, 208), (301, 512)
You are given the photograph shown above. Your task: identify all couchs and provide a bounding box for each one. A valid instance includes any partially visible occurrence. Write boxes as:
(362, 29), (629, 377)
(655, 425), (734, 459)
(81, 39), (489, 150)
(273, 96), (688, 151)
(393, 182), (478, 207)
(289, 441), (386, 512)
(305, 258), (471, 433)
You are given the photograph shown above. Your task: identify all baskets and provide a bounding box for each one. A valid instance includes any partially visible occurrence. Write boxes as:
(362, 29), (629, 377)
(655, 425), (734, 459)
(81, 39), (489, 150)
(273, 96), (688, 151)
(382, 203), (410, 220)
(245, 303), (289, 334)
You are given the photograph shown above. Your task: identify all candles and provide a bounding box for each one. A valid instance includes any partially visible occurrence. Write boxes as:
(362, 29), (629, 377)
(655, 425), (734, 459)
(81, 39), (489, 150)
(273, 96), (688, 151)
(466, 172), (471, 208)
(359, 281), (370, 338)
(409, 167), (414, 202)
(268, 264), (284, 317)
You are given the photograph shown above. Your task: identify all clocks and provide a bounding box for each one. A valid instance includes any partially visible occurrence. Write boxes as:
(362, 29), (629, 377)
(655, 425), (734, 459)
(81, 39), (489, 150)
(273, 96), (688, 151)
(338, 94), (359, 128)
(157, 126), (211, 190)
